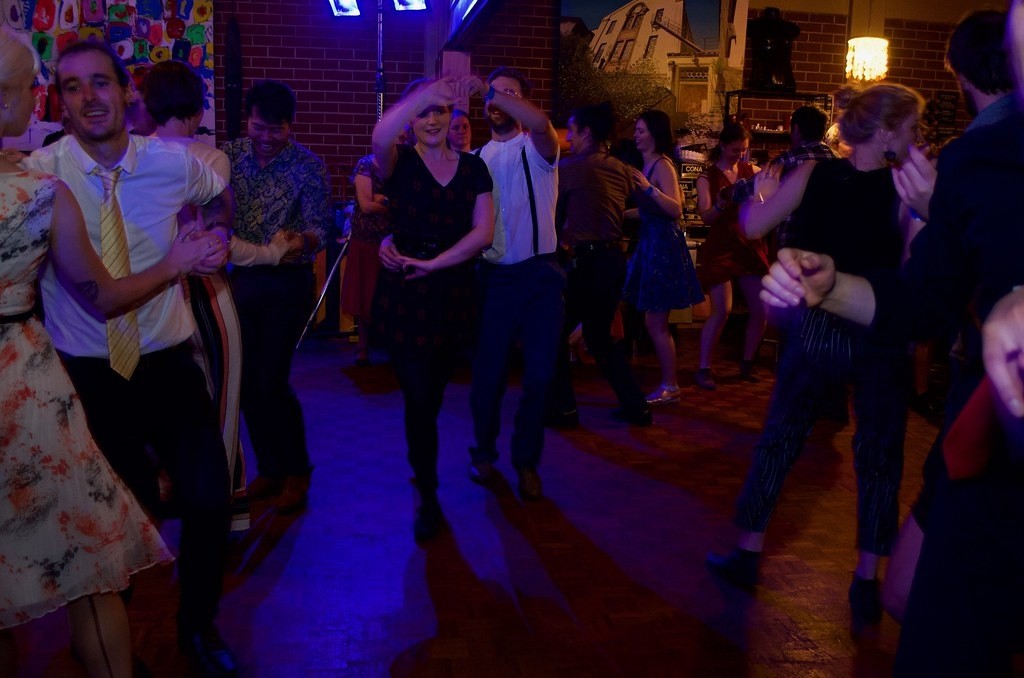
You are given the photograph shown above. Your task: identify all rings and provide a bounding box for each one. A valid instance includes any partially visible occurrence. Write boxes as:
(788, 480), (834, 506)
(176, 234), (183, 240)
(766, 173), (771, 176)
(207, 242), (212, 248)
(215, 239), (220, 244)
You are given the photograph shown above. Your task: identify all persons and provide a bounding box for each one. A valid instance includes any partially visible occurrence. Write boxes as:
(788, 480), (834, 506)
(0, 29), (335, 678)
(345, 67), (704, 498)
(696, 0), (1024, 678)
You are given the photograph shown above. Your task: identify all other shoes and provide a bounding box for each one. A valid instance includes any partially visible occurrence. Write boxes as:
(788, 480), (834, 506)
(644, 386), (682, 405)
(547, 410), (581, 428)
(182, 630), (246, 678)
(472, 473), (510, 499)
(697, 369), (716, 389)
(519, 468), (542, 498)
(269, 494), (308, 514)
(235, 475), (273, 502)
(740, 366), (758, 384)
(414, 508), (444, 543)
(706, 546), (759, 589)
(848, 573), (885, 628)
(611, 403), (654, 427)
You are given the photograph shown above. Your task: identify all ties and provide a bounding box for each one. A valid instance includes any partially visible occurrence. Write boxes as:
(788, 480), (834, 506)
(96, 170), (140, 380)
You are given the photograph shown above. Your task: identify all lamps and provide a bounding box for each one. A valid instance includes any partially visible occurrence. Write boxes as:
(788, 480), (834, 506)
(845, 0), (889, 79)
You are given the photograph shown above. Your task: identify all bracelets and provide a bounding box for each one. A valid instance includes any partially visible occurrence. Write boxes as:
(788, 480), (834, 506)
(715, 203), (721, 211)
(302, 236), (310, 259)
(206, 220), (227, 232)
(644, 184), (654, 196)
(908, 208), (924, 221)
(483, 85), (495, 101)
